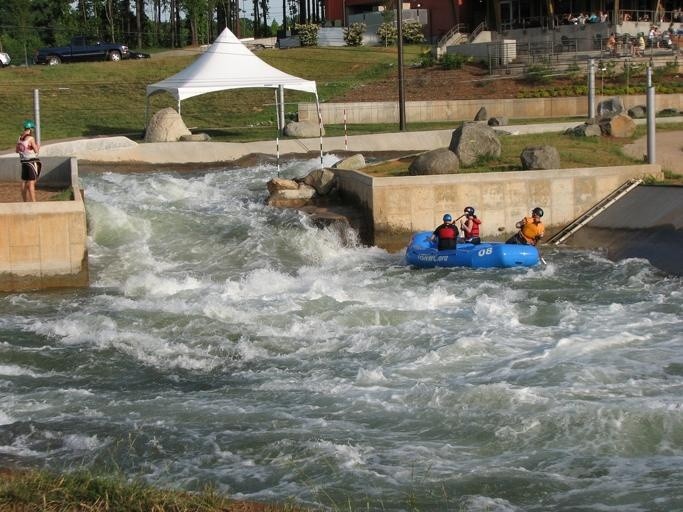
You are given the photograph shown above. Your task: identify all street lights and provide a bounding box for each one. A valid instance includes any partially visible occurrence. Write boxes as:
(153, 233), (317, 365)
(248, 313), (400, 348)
(417, 4), (420, 21)
(598, 63), (606, 112)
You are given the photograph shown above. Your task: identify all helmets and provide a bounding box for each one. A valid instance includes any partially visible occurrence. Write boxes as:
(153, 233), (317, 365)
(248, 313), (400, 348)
(443, 214), (452, 221)
(23, 119), (36, 128)
(464, 207), (474, 214)
(532, 208), (543, 216)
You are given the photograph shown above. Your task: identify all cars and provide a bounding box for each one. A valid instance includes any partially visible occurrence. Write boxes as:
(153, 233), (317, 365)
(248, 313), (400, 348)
(0, 53), (11, 68)
(128, 51), (150, 59)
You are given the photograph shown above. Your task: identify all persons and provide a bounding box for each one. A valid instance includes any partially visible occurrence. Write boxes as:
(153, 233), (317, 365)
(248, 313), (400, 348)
(505, 207), (545, 245)
(559, 4), (683, 58)
(16, 120), (40, 202)
(430, 214), (459, 250)
(460, 207), (481, 244)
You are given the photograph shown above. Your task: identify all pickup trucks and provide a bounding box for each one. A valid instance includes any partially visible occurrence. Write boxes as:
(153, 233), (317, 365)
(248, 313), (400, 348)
(33, 36), (128, 65)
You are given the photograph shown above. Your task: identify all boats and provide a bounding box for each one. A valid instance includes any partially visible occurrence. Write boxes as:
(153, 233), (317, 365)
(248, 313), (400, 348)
(406, 232), (538, 269)
